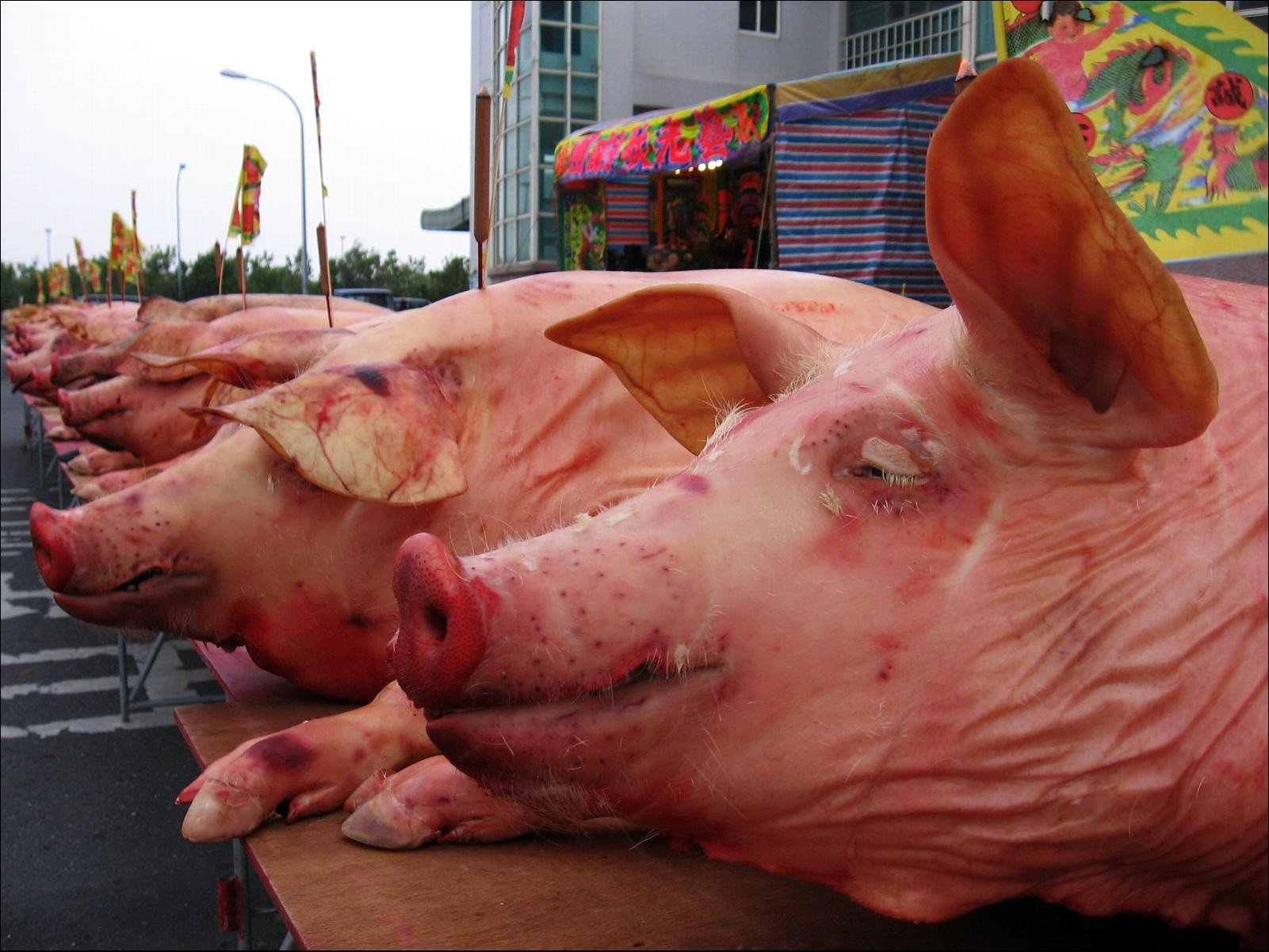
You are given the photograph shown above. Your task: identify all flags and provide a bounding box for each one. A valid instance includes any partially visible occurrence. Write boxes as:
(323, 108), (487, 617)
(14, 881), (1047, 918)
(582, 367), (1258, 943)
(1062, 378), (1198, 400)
(228, 147), (268, 243)
(37, 215), (141, 303)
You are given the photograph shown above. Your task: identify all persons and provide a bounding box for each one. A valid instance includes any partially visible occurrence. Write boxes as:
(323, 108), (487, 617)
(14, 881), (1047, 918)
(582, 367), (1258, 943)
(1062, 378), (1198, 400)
(710, 219), (738, 270)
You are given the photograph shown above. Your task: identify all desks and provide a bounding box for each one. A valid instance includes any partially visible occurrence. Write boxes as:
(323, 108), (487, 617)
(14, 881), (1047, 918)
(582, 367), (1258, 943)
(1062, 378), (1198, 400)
(179, 703), (956, 951)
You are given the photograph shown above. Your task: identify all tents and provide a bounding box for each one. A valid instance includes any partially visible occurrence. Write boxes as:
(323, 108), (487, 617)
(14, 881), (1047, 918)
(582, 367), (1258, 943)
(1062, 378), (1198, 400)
(552, 51), (976, 312)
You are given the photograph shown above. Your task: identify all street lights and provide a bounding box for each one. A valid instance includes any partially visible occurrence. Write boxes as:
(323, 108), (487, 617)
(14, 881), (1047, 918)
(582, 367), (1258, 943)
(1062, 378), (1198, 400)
(176, 163), (188, 301)
(219, 69), (310, 298)
(44, 224), (52, 275)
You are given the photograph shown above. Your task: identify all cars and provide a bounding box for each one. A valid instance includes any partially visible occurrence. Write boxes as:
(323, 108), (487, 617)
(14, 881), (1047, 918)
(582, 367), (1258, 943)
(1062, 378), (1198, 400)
(73, 294), (150, 306)
(333, 289), (433, 314)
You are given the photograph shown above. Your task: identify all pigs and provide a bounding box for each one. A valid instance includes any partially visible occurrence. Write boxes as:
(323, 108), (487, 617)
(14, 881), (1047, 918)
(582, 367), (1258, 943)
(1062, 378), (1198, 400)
(32, 270), (945, 842)
(57, 307), (420, 501)
(342, 57), (1268, 943)
(46, 294), (395, 440)
(4, 300), (147, 406)
